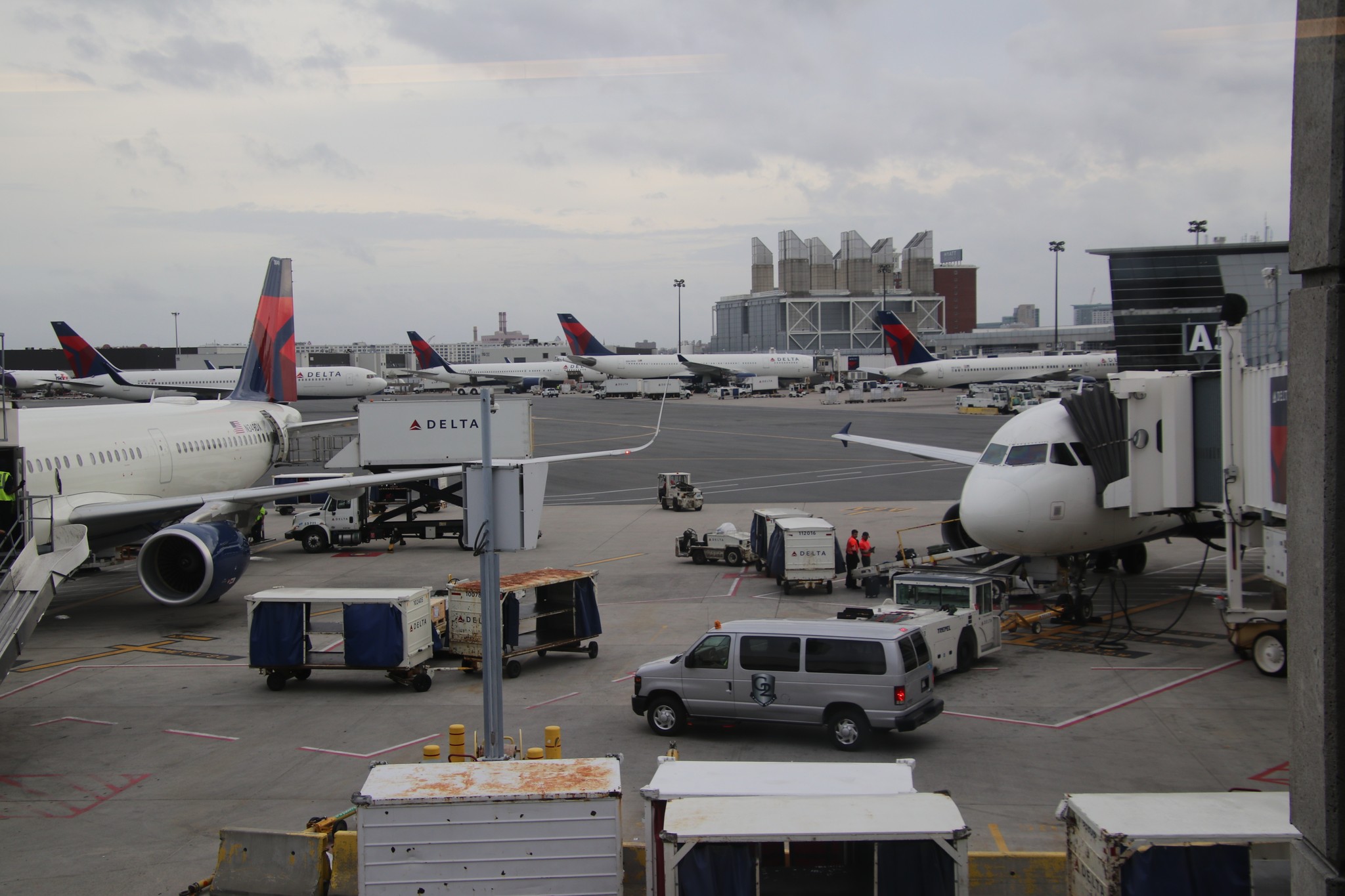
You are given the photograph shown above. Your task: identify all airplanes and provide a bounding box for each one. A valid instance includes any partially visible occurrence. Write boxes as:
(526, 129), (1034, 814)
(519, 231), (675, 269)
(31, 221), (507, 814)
(1, 257), (1255, 684)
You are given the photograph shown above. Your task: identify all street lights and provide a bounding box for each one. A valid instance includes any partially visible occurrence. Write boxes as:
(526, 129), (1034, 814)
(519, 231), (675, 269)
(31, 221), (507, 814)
(1049, 240), (1067, 351)
(1187, 220), (1208, 246)
(878, 263), (892, 356)
(171, 312), (180, 355)
(673, 279), (686, 354)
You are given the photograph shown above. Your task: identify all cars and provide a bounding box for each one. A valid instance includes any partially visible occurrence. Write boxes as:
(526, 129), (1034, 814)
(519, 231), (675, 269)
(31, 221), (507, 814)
(877, 381), (889, 391)
(533, 390), (541, 396)
(1011, 400), (1040, 416)
(457, 385), (481, 395)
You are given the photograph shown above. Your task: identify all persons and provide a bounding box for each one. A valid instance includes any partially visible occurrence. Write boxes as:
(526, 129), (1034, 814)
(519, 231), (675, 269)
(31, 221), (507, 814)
(844, 529), (862, 590)
(859, 531), (875, 585)
(250, 507), (265, 544)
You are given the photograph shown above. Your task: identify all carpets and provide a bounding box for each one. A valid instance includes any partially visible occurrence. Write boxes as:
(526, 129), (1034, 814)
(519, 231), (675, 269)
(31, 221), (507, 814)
(330, 552), (385, 557)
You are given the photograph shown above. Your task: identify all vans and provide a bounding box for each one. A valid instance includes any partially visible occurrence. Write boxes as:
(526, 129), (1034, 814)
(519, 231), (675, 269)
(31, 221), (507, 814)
(631, 618), (945, 753)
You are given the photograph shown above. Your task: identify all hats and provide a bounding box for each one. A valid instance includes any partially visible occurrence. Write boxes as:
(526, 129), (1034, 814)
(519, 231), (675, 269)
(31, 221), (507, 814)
(863, 531), (871, 538)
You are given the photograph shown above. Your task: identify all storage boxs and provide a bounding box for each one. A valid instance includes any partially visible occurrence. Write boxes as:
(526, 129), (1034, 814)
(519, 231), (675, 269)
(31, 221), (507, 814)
(430, 599), (445, 622)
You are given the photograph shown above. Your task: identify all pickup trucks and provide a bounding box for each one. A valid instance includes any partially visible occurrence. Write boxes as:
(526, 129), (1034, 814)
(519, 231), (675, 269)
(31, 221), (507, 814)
(845, 379), (859, 389)
(383, 386), (395, 395)
(880, 381), (900, 391)
(815, 380), (845, 394)
(542, 388), (559, 398)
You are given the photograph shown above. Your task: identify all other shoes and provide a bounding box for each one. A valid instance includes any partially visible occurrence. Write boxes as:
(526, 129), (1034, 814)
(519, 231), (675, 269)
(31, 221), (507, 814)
(846, 584), (862, 589)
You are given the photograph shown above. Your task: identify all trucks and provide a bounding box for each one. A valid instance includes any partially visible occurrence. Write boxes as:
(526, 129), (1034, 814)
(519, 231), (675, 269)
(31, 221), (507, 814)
(592, 377), (643, 399)
(641, 378), (693, 401)
(413, 377), (450, 394)
(736, 375), (779, 396)
(720, 381), (737, 388)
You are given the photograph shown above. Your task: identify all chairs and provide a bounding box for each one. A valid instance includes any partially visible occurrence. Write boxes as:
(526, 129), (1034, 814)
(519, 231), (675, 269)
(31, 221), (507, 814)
(695, 533), (712, 545)
